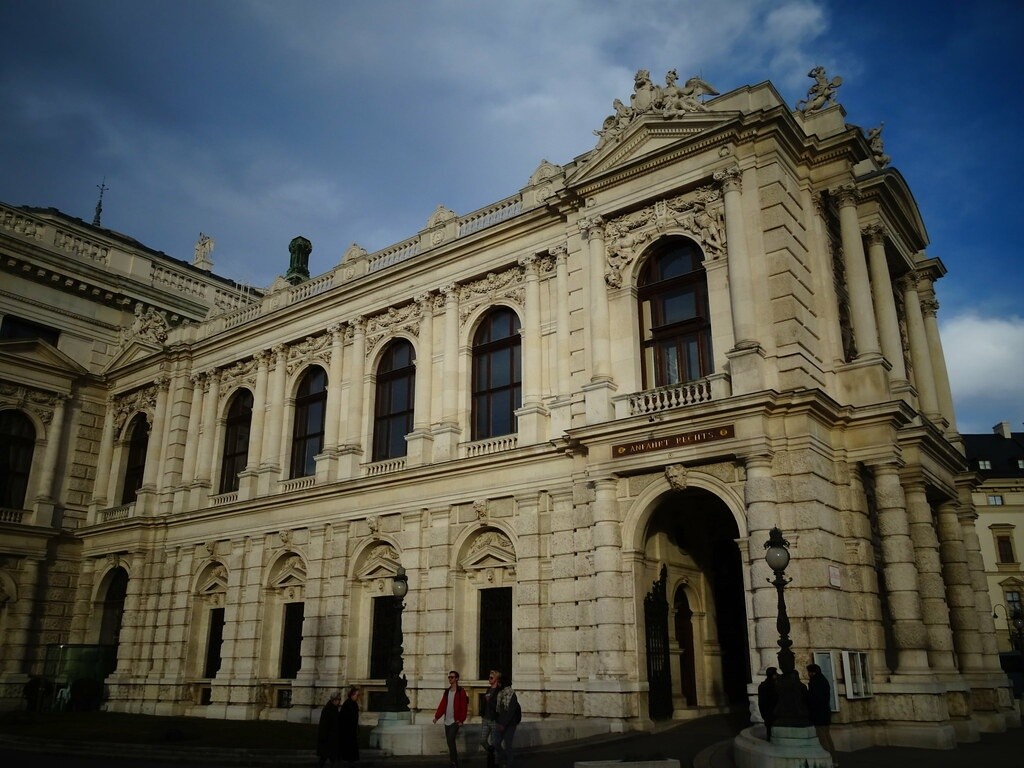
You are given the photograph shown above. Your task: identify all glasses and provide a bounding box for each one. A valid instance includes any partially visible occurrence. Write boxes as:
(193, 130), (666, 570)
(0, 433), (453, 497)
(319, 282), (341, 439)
(448, 676), (456, 679)
(489, 676), (493, 680)
(336, 697), (341, 701)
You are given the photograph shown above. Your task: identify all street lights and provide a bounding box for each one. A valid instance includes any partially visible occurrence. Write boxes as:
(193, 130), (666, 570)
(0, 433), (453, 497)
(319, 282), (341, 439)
(384, 571), (410, 711)
(993, 604), (1012, 651)
(762, 524), (812, 727)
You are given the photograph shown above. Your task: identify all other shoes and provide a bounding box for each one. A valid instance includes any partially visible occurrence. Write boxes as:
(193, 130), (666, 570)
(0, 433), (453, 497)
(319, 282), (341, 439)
(450, 762), (458, 768)
(502, 763), (507, 768)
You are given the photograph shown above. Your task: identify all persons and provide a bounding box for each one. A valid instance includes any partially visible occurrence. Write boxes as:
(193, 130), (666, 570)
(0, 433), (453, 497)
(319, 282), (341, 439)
(808, 66), (830, 97)
(662, 72), (713, 118)
(433, 671), (468, 768)
(758, 667), (779, 741)
(133, 311), (166, 343)
(481, 671), (519, 768)
(867, 120), (891, 169)
(194, 236), (214, 262)
(592, 100), (636, 150)
(316, 688), (360, 768)
(807, 664), (839, 767)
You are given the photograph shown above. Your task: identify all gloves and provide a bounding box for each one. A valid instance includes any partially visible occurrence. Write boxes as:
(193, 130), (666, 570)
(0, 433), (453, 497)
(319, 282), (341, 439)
(496, 725), (504, 732)
